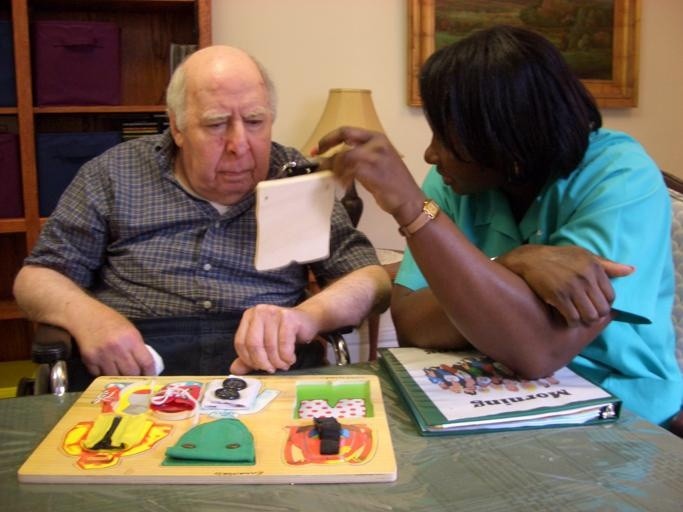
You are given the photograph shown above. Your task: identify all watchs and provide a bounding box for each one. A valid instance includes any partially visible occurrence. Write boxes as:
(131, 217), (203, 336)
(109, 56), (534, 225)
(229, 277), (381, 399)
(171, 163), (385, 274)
(399, 199), (442, 238)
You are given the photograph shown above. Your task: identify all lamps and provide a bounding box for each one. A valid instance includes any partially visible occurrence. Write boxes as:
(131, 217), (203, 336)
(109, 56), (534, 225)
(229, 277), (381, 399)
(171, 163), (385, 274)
(299, 89), (405, 227)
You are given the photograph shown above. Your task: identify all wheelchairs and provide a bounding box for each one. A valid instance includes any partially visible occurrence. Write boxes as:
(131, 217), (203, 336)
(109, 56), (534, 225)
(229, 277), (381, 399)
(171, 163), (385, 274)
(16, 323), (353, 398)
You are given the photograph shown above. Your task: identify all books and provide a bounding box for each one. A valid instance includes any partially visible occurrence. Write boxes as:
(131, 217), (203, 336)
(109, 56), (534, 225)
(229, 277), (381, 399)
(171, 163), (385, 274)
(377, 334), (624, 436)
(122, 112), (170, 142)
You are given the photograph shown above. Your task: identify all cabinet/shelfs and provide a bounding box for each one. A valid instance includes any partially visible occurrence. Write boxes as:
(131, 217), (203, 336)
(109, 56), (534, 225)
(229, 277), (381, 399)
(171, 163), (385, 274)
(0, 0), (213, 358)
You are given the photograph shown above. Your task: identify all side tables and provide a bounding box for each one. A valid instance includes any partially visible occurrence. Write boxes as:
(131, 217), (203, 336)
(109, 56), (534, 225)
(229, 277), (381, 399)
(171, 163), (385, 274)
(309, 245), (405, 359)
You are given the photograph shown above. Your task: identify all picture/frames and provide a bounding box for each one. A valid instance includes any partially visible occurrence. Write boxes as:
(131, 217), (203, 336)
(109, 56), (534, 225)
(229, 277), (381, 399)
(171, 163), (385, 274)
(406, 0), (640, 108)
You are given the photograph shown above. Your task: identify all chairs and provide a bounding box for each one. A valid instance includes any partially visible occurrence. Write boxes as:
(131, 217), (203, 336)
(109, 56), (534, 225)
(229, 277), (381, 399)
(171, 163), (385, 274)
(662, 170), (683, 378)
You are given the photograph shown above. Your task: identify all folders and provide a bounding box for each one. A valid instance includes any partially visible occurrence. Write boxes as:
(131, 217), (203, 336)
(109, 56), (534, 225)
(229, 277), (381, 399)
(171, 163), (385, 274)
(375, 347), (623, 437)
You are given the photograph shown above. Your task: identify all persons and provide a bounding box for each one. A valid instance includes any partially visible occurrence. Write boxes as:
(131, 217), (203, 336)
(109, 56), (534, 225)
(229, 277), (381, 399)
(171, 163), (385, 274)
(12, 44), (394, 376)
(312, 26), (682, 426)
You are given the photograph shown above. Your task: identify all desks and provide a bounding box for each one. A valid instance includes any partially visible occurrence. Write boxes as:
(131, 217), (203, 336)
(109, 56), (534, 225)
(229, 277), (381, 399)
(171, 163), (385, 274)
(1, 362), (683, 511)
